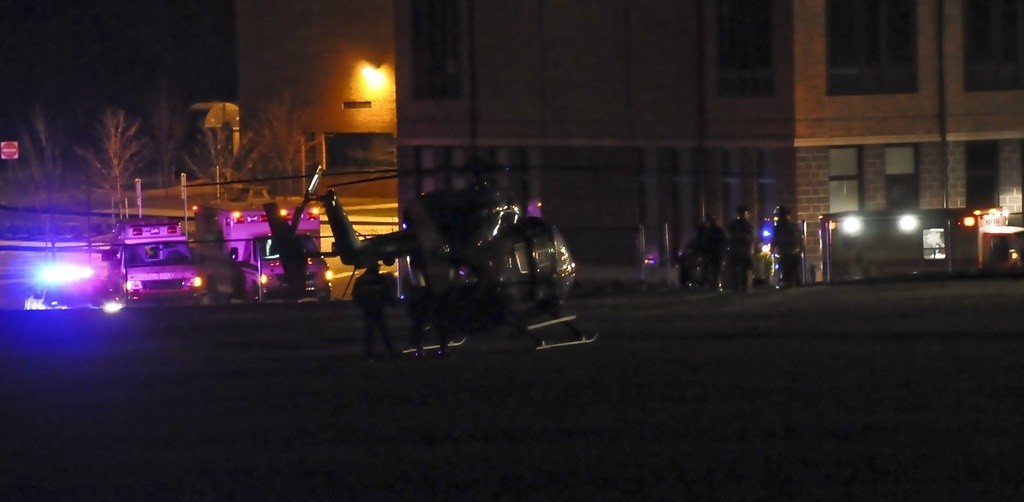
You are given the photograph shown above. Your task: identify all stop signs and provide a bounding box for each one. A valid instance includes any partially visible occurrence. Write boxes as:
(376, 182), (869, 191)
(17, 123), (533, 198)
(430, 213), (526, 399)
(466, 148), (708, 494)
(1, 141), (19, 160)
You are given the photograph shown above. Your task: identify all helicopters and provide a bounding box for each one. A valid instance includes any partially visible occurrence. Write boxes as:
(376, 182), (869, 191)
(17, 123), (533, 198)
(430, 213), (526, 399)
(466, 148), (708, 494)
(180, 158), (781, 360)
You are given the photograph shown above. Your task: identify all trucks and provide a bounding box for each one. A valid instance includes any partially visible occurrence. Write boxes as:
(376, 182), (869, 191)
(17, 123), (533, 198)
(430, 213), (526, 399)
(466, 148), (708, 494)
(818, 207), (1024, 287)
(192, 202), (332, 306)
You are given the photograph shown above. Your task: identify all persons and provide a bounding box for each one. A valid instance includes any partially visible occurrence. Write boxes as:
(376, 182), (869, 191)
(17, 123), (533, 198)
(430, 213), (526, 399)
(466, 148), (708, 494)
(353, 263), (396, 361)
(681, 222), (726, 289)
(403, 257), (449, 360)
(729, 207), (756, 293)
(771, 206), (804, 288)
(148, 246), (160, 259)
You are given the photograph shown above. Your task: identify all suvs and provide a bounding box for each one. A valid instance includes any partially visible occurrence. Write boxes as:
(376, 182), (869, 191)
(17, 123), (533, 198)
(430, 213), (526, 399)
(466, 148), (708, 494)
(108, 216), (207, 304)
